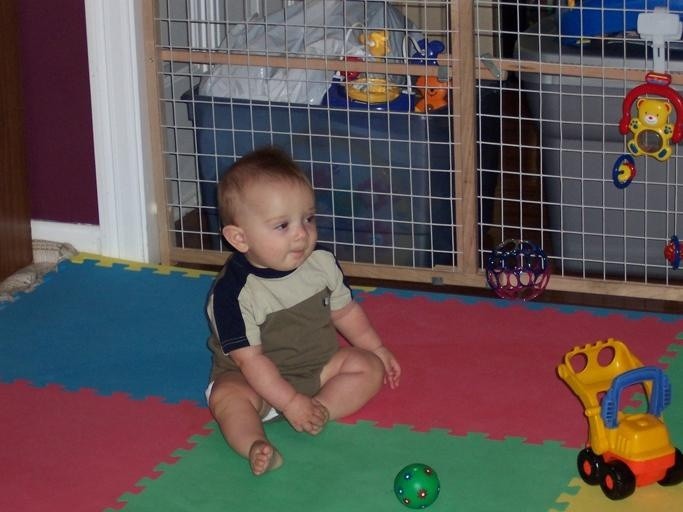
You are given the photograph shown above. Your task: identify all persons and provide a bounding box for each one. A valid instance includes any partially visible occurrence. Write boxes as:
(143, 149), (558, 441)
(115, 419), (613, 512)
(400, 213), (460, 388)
(200, 144), (401, 476)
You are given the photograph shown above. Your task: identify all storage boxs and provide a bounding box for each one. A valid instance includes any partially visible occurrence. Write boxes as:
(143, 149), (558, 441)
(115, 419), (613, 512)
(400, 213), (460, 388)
(510, 27), (682, 289)
(176, 72), (507, 271)
